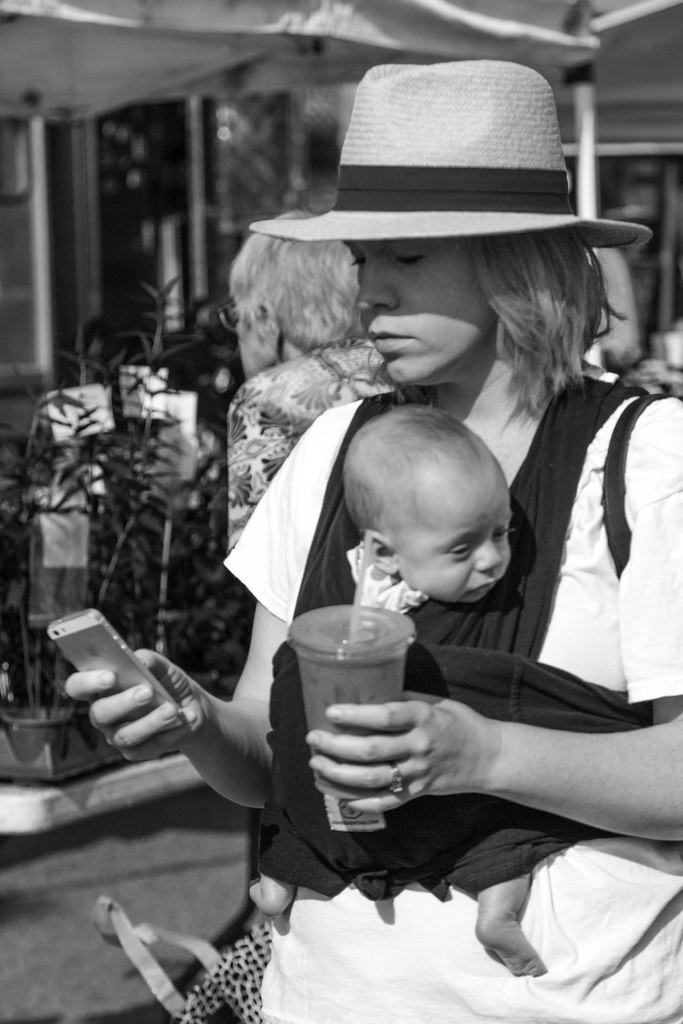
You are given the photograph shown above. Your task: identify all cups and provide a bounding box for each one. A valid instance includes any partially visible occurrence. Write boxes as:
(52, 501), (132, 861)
(286, 605), (417, 795)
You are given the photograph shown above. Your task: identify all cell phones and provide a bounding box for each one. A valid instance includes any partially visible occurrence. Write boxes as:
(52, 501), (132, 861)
(47, 609), (183, 734)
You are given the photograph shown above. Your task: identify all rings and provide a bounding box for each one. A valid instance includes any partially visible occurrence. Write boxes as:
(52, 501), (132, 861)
(387, 763), (403, 793)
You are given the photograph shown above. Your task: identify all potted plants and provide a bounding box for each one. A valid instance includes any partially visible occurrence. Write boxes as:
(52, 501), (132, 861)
(0, 275), (243, 786)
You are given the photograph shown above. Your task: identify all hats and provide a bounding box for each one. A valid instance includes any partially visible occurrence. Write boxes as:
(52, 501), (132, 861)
(247, 60), (651, 249)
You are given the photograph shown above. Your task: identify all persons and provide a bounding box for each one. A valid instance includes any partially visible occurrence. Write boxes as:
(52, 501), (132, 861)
(249, 407), (547, 977)
(65, 60), (683, 1024)
(227, 210), (396, 549)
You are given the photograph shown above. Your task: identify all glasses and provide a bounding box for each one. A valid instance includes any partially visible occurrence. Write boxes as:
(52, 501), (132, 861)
(217, 303), (243, 332)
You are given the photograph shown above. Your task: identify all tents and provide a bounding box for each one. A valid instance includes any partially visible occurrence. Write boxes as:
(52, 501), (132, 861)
(0, 0), (683, 365)
